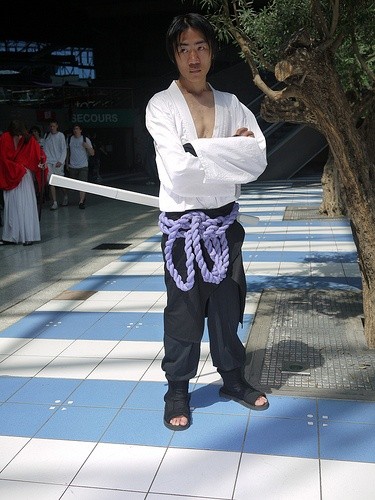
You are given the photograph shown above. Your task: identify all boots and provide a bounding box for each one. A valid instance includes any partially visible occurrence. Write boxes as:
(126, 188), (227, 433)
(164, 382), (191, 431)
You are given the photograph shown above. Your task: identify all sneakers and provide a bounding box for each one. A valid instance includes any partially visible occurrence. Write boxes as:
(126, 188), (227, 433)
(51, 203), (58, 210)
(63, 198), (68, 205)
(79, 201), (84, 209)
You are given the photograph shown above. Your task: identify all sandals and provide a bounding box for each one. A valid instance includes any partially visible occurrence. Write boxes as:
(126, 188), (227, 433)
(218, 369), (270, 411)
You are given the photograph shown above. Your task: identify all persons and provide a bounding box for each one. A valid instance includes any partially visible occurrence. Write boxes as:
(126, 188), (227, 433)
(145, 12), (269, 431)
(0, 114), (48, 246)
(31, 120), (102, 210)
